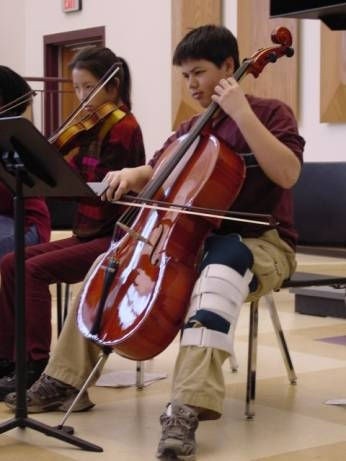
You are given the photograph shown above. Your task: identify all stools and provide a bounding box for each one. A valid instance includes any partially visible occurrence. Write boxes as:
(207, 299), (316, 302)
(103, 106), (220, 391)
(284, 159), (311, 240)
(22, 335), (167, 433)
(137, 286), (296, 418)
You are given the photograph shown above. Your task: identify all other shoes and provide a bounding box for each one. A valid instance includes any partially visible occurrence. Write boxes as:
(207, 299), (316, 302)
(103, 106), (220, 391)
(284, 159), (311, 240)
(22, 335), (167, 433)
(5, 373), (95, 413)
(0, 365), (43, 401)
(156, 401), (199, 461)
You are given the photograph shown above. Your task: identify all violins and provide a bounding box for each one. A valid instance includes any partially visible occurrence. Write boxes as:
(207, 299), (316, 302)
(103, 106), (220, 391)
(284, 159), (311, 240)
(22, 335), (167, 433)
(51, 98), (120, 155)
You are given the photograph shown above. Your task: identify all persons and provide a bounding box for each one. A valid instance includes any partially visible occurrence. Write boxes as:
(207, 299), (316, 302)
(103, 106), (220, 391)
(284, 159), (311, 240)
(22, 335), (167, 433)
(0, 66), (51, 257)
(4, 25), (306, 461)
(0, 46), (147, 403)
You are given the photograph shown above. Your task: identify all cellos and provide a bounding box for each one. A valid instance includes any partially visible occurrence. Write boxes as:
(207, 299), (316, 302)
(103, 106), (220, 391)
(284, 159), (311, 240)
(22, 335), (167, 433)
(53, 26), (295, 435)
